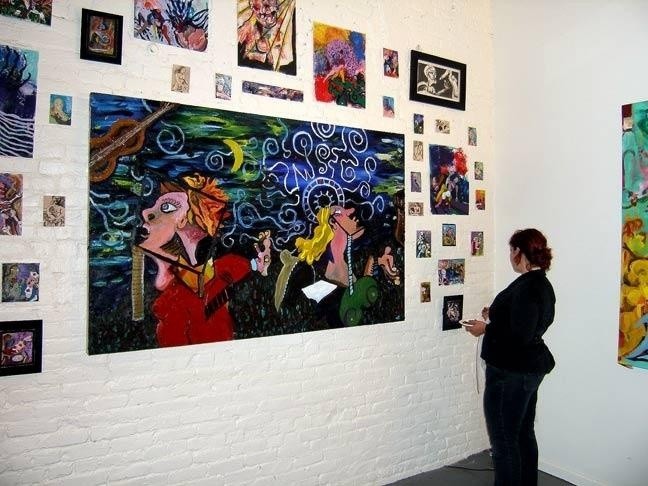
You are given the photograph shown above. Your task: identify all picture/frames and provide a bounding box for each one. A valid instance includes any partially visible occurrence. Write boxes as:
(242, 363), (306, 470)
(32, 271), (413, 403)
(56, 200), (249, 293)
(0, 320), (43, 376)
(79, 4), (123, 66)
(409, 49), (467, 112)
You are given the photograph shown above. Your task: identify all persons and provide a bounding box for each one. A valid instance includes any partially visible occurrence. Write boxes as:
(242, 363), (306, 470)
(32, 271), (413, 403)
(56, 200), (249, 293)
(462, 227), (557, 486)
(443, 226), (455, 245)
(472, 236), (480, 255)
(172, 66), (188, 91)
(439, 261), (463, 285)
(410, 203), (421, 215)
(421, 283), (429, 302)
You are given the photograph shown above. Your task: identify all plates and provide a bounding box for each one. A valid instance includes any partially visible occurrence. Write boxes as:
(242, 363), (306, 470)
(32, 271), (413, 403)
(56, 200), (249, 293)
(459, 318), (489, 328)
